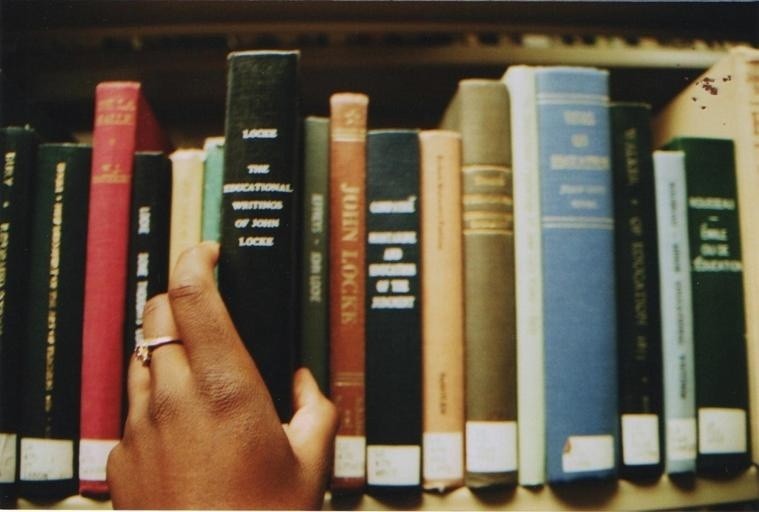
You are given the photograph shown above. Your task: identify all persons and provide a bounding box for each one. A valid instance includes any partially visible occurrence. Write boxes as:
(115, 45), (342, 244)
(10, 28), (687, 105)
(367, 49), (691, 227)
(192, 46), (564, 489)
(102, 241), (339, 510)
